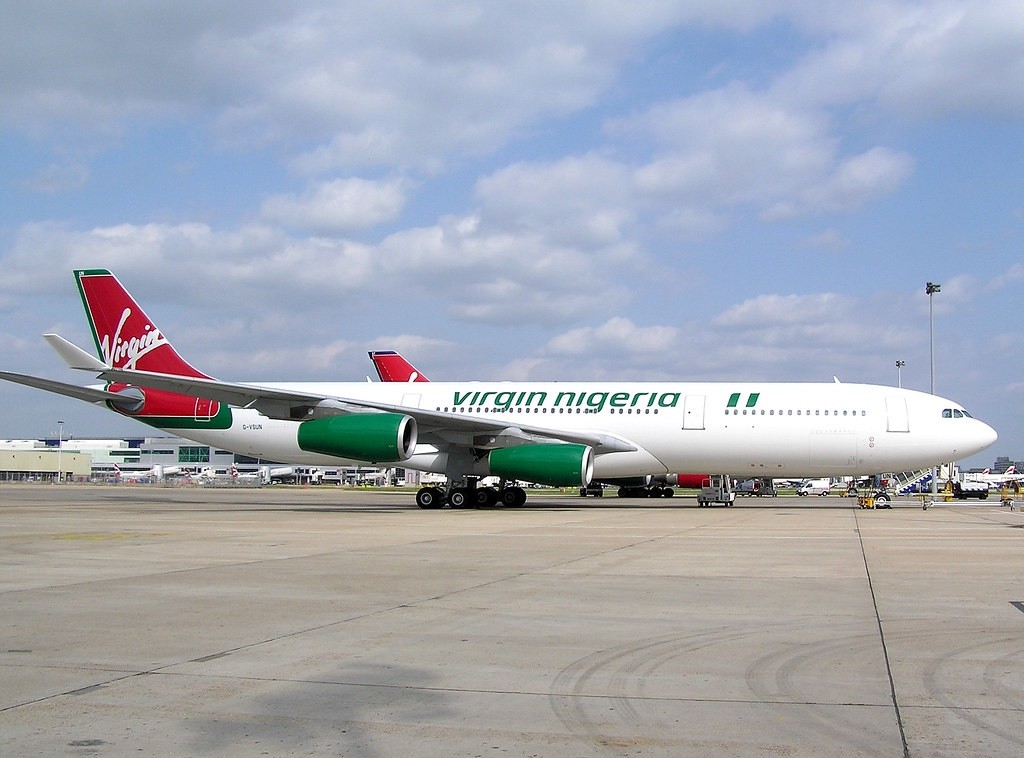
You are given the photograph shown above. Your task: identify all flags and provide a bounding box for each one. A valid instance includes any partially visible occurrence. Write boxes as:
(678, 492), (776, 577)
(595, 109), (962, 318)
(230, 464), (239, 478)
(1004, 465), (1015, 474)
(982, 468), (990, 474)
(184, 466), (190, 479)
(114, 463), (120, 477)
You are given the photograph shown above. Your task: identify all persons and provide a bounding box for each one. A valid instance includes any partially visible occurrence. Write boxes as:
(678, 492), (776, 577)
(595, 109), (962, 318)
(1010, 500), (1016, 511)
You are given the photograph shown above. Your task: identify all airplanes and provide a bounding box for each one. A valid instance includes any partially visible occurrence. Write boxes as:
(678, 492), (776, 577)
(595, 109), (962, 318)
(974, 465), (1024, 488)
(0, 265), (999, 511)
(229, 462), (293, 484)
(111, 462), (180, 481)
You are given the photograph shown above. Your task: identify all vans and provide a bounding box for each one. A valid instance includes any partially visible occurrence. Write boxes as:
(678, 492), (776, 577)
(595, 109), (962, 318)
(795, 479), (830, 496)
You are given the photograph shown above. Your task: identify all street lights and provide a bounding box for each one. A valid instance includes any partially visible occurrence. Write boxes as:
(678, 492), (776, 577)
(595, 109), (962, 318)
(56, 421), (64, 483)
(895, 359), (905, 386)
(925, 281), (942, 393)
